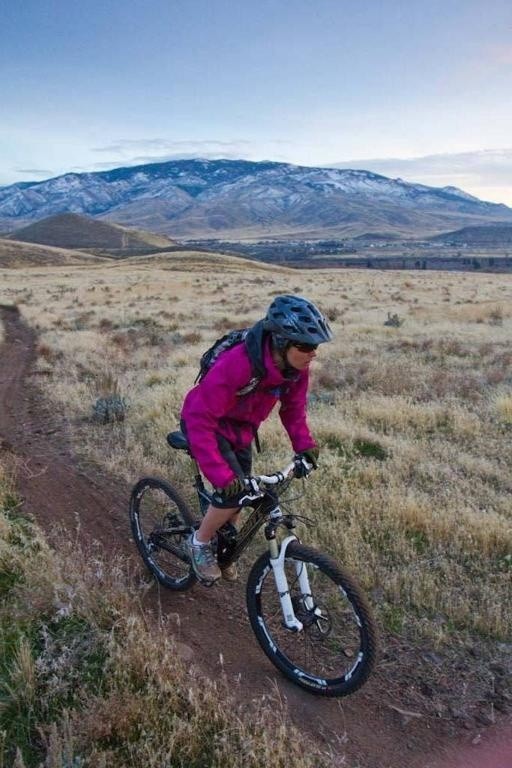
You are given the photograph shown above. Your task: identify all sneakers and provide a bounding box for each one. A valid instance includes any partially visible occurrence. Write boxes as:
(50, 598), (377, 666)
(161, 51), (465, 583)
(184, 531), (222, 582)
(219, 547), (241, 581)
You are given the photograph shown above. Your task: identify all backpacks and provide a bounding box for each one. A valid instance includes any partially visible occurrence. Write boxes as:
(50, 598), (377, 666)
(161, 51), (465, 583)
(199, 329), (264, 398)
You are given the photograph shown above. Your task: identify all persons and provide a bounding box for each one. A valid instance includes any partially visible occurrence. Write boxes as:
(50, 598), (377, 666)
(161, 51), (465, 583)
(179, 295), (335, 582)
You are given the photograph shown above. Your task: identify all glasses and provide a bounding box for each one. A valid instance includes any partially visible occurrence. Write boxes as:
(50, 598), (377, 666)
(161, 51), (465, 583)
(289, 342), (319, 353)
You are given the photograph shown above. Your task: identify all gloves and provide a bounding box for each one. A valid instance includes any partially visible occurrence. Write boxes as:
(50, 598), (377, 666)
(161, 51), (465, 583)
(215, 478), (244, 497)
(296, 447), (322, 471)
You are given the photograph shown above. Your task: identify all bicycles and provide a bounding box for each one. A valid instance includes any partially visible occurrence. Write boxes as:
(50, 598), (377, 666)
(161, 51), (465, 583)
(130, 429), (378, 698)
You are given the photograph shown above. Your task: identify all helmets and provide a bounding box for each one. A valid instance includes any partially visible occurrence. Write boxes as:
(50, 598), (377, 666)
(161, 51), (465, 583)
(259, 293), (336, 351)
